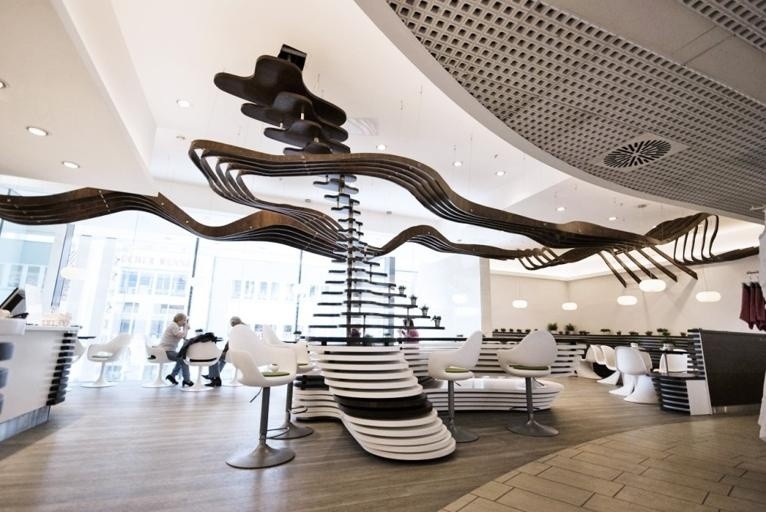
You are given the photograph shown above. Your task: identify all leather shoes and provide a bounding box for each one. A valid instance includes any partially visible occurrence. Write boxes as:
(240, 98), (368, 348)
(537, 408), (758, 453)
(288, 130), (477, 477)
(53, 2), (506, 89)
(201, 375), (221, 386)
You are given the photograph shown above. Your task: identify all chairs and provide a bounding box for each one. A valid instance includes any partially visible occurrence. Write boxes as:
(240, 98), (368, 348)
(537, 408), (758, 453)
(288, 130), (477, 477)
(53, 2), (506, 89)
(70, 326), (313, 469)
(427, 331), (484, 443)
(577, 344), (695, 405)
(498, 330), (561, 437)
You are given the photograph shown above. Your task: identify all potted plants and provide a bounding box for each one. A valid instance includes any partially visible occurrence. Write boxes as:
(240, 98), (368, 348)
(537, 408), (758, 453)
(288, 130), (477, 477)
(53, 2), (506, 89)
(432, 315), (441, 327)
(410, 294), (417, 304)
(421, 305), (428, 316)
(548, 322), (670, 336)
(399, 285), (405, 294)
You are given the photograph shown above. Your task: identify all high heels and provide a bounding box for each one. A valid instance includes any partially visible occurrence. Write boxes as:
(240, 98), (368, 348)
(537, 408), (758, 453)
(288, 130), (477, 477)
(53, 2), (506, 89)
(166, 375), (193, 386)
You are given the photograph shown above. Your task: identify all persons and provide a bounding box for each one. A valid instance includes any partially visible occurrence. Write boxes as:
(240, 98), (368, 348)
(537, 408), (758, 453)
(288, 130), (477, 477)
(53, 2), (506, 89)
(399, 318), (420, 366)
(198, 315), (246, 387)
(154, 312), (194, 390)
